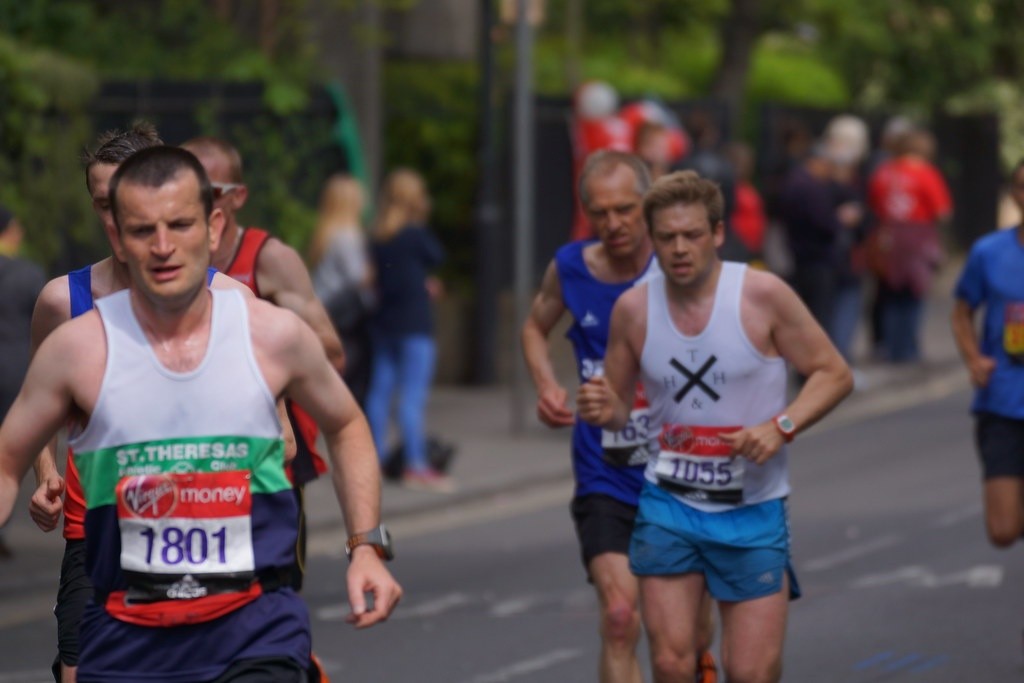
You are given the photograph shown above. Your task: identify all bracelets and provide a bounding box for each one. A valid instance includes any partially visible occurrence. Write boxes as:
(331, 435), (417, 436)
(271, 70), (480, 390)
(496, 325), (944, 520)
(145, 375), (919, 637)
(345, 522), (394, 561)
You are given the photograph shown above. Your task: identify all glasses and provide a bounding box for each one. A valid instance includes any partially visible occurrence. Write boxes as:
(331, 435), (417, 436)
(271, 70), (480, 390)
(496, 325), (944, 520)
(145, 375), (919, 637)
(210, 183), (243, 200)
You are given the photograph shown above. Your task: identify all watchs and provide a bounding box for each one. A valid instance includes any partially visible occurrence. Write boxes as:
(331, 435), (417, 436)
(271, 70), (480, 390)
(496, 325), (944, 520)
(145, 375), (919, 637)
(772, 413), (795, 443)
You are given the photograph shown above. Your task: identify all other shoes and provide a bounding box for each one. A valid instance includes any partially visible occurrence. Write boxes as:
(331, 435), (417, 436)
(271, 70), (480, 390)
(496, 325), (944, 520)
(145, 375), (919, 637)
(405, 466), (458, 494)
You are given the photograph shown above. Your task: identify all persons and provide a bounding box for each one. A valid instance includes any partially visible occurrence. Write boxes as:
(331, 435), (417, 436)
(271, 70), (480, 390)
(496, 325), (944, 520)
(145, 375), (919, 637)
(1, 146), (402, 683)
(583, 82), (950, 364)
(576, 168), (852, 683)
(951, 159), (1024, 548)
(315, 171), (376, 397)
(522, 149), (719, 683)
(179, 137), (347, 683)
(369, 167), (458, 491)
(30, 123), (163, 683)
(0, 207), (48, 424)
